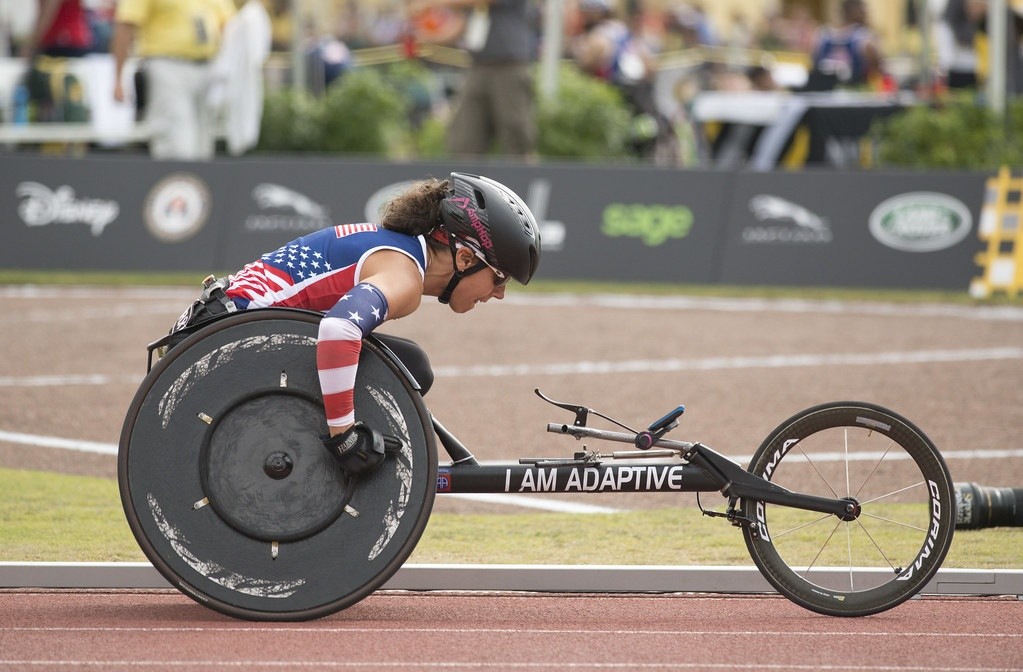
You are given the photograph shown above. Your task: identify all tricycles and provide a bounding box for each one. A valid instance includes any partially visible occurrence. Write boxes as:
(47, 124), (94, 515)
(114, 305), (957, 624)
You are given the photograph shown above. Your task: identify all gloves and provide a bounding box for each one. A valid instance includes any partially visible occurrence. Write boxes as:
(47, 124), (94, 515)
(319, 422), (402, 479)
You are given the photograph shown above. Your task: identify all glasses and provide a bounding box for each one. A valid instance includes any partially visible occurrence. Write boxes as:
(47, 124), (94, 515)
(438, 224), (510, 288)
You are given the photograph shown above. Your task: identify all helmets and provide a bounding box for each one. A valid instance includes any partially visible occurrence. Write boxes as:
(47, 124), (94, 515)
(442, 171), (542, 286)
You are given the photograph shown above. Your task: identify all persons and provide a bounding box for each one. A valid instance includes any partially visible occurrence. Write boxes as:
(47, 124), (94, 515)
(560, 0), (1023, 172)
(25, 0), (272, 158)
(305, 0), (543, 158)
(165, 172), (542, 473)
